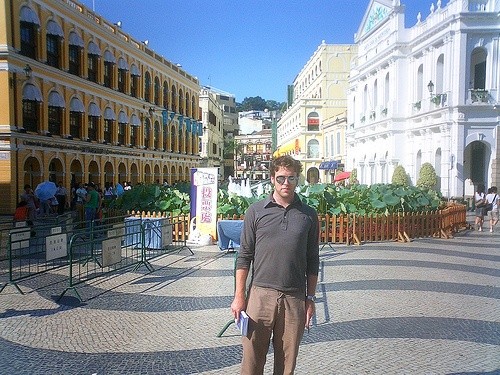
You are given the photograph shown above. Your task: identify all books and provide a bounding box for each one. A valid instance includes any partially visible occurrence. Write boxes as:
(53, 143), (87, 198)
(234, 311), (249, 336)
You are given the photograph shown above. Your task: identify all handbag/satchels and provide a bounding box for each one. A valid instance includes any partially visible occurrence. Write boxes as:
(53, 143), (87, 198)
(485, 203), (493, 211)
(475, 199), (485, 208)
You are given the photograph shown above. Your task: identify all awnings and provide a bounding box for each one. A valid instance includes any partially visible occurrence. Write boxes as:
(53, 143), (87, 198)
(19, 6), (143, 77)
(21, 84), (141, 126)
(319, 160), (340, 170)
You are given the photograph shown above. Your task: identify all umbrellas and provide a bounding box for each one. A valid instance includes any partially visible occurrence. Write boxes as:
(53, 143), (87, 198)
(333, 170), (352, 185)
(36, 181), (57, 200)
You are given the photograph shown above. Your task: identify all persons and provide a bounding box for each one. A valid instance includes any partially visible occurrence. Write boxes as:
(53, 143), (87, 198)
(51, 179), (192, 217)
(475, 183), (486, 232)
(485, 186), (499, 233)
(22, 184), (38, 220)
(229, 155), (322, 375)
(14, 202), (37, 239)
(76, 182), (101, 239)
(39, 198), (51, 216)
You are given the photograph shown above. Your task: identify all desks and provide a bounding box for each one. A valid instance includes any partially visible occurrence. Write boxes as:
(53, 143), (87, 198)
(218, 220), (246, 255)
(124, 216), (173, 251)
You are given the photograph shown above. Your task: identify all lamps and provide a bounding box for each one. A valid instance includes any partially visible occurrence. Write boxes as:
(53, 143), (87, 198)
(427, 79), (448, 108)
(9, 64), (34, 89)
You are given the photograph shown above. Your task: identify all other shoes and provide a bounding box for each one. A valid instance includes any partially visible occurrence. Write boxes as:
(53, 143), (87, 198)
(475, 223), (497, 233)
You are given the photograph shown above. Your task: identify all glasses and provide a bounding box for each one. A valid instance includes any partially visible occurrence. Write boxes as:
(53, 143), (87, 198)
(276, 175), (299, 186)
